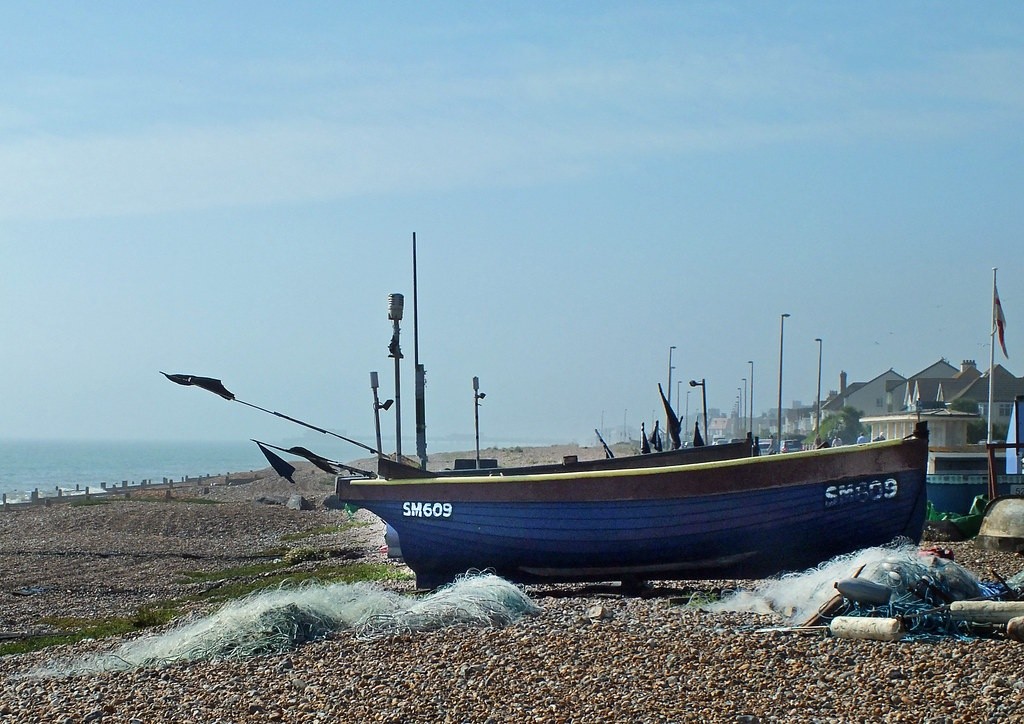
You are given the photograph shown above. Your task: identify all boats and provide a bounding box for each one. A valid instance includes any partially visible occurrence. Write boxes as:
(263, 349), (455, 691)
(377, 433), (754, 480)
(927, 474), (1024, 540)
(336, 422), (928, 591)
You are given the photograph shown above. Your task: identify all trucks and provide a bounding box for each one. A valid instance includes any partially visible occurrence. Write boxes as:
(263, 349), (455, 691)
(712, 434), (726, 445)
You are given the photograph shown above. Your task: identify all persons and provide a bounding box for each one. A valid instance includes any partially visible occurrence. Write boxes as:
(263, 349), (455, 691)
(814, 434), (821, 449)
(766, 434), (776, 455)
(832, 435), (842, 447)
(857, 432), (869, 444)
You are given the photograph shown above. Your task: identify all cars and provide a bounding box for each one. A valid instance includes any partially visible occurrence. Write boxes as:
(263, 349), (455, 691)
(758, 442), (771, 456)
(780, 440), (800, 453)
(716, 439), (729, 445)
(679, 441), (693, 449)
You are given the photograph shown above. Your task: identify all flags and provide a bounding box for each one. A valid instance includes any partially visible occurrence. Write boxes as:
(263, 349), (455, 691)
(285, 446), (339, 475)
(640, 427), (651, 454)
(162, 373), (235, 400)
(660, 387), (682, 449)
(603, 442), (614, 458)
(993, 281), (1009, 360)
(258, 443), (296, 484)
(650, 425), (663, 452)
(693, 422), (705, 447)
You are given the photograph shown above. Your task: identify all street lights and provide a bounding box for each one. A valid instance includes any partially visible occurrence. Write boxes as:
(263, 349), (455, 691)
(666, 346), (675, 450)
(685, 392), (691, 441)
(690, 379), (708, 446)
(677, 381), (682, 422)
(814, 338), (822, 436)
(742, 379), (746, 438)
(748, 361), (754, 434)
(778, 313), (790, 454)
(733, 388), (741, 435)
